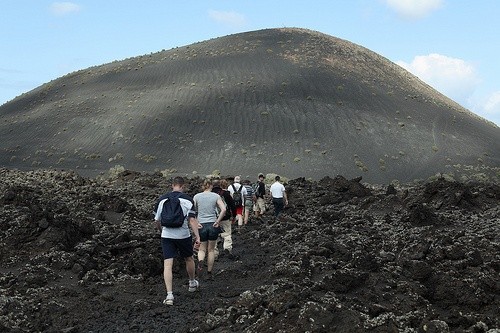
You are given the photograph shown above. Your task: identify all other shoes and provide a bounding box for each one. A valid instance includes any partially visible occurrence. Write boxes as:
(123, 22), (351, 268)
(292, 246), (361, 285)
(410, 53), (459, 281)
(214, 256), (220, 262)
(205, 274), (212, 280)
(197, 260), (204, 278)
(238, 232), (242, 234)
(243, 226), (247, 229)
(224, 249), (235, 260)
(231, 229), (236, 234)
(254, 216), (264, 223)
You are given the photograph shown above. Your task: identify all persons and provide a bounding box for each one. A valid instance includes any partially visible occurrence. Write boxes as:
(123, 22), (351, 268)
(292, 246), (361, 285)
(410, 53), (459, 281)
(211, 178), (236, 259)
(228, 176), (248, 236)
(242, 180), (256, 226)
(269, 175), (288, 219)
(252, 174), (266, 220)
(194, 178), (227, 281)
(150, 176), (200, 306)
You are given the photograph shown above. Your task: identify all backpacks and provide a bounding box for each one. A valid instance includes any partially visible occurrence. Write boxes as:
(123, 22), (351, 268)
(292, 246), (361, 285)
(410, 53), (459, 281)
(231, 184), (243, 208)
(160, 192), (187, 228)
(216, 190), (229, 216)
(252, 181), (261, 196)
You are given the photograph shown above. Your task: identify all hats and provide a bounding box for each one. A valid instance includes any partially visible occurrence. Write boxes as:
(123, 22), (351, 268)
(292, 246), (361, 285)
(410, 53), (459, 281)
(259, 175), (266, 179)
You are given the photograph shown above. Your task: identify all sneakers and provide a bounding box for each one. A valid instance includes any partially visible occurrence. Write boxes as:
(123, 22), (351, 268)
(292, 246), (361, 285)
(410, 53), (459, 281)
(188, 280), (199, 291)
(163, 297), (174, 305)
